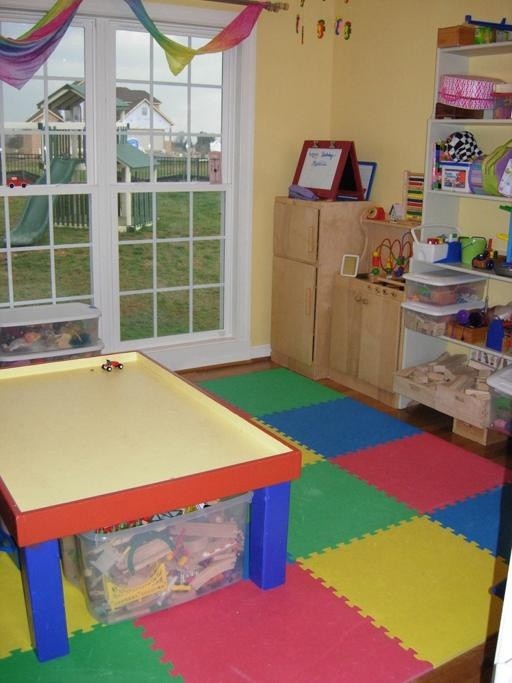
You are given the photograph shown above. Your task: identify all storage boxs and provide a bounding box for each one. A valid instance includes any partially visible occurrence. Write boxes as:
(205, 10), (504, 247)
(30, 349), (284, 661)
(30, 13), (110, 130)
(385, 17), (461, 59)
(75, 490), (255, 624)
(0, 300), (101, 355)
(0, 339), (111, 370)
(401, 301), (479, 337)
(401, 269), (487, 308)
(487, 367), (511, 429)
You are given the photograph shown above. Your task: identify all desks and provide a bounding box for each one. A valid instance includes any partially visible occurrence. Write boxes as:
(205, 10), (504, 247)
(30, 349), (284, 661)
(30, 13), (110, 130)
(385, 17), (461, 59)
(0, 349), (302, 663)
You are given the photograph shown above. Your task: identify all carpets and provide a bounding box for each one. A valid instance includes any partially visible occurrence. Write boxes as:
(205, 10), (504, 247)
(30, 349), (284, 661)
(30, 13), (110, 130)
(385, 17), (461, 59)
(0, 367), (511, 682)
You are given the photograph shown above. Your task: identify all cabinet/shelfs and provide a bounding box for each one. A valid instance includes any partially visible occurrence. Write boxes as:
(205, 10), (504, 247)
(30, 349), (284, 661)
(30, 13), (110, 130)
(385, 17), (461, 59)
(270, 196), (362, 382)
(400, 15), (512, 447)
(328, 206), (421, 409)
